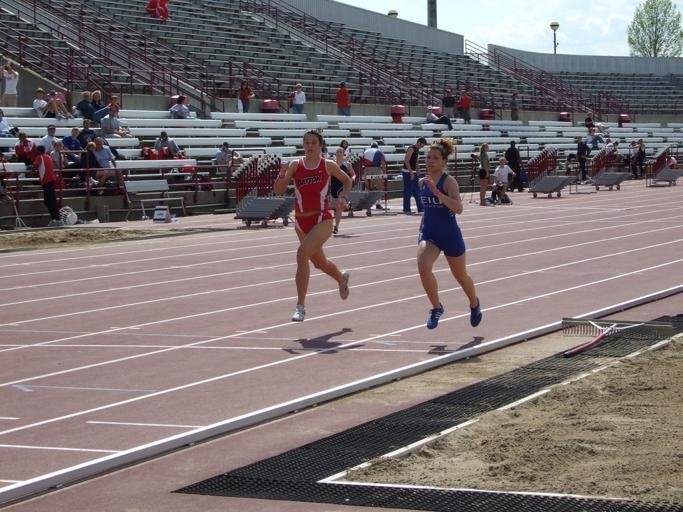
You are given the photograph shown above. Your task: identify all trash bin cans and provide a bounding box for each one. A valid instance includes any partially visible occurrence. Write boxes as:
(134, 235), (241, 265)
(260, 99), (280, 113)
(620, 114), (632, 123)
(432, 106), (442, 118)
(46, 91), (67, 106)
(479, 109), (495, 120)
(560, 112), (573, 122)
(169, 94), (191, 110)
(391, 104), (406, 117)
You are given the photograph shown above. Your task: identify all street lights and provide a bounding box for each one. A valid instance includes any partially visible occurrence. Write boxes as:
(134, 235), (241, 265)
(388, 9), (399, 19)
(549, 22), (562, 54)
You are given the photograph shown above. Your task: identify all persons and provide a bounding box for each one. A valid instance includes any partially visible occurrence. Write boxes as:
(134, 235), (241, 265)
(666, 156), (677, 185)
(169, 96), (192, 120)
(417, 140), (482, 329)
(274, 130), (386, 321)
(239, 80), (255, 113)
(402, 137), (427, 214)
(577, 113), (645, 183)
(479, 140), (525, 206)
(290, 84), (306, 121)
(336, 81), (350, 116)
(216, 142), (241, 172)
(511, 93), (519, 121)
(154, 131), (180, 157)
(426, 90), (472, 132)
(0, 62), (132, 226)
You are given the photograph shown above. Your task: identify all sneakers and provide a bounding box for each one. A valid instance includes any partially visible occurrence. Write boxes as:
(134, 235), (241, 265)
(427, 304), (444, 329)
(339, 273), (349, 300)
(292, 304), (305, 322)
(470, 297), (481, 326)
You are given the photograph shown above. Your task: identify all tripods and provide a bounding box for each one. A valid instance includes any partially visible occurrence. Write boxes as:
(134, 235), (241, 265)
(461, 164), (498, 203)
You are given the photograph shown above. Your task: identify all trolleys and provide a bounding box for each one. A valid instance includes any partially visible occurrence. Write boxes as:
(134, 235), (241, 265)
(520, 140), (573, 199)
(643, 143), (681, 187)
(230, 149), (292, 227)
(323, 147), (390, 218)
(580, 141), (625, 192)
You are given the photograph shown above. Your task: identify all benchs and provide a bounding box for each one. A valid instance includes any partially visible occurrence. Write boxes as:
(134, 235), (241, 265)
(0, 2), (682, 230)
